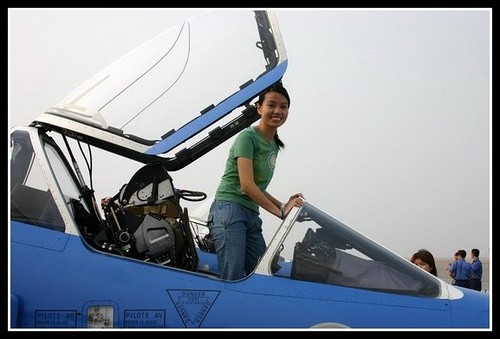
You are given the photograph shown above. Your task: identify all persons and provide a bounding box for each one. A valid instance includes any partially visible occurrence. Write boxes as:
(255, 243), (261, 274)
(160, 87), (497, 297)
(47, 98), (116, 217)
(208, 84), (306, 286)
(470, 249), (482, 291)
(411, 250), (437, 277)
(448, 250), (474, 289)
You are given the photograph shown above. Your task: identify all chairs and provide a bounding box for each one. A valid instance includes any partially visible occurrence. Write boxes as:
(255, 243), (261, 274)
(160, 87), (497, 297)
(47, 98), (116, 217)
(11, 184), (65, 233)
(179, 207), (221, 278)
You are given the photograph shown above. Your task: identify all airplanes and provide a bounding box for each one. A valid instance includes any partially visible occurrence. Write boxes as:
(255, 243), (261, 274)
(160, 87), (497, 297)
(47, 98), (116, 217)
(11, 7), (490, 329)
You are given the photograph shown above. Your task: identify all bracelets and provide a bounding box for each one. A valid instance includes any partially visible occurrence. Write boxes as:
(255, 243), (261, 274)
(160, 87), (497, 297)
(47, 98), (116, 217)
(281, 202), (288, 209)
(281, 209), (285, 220)
